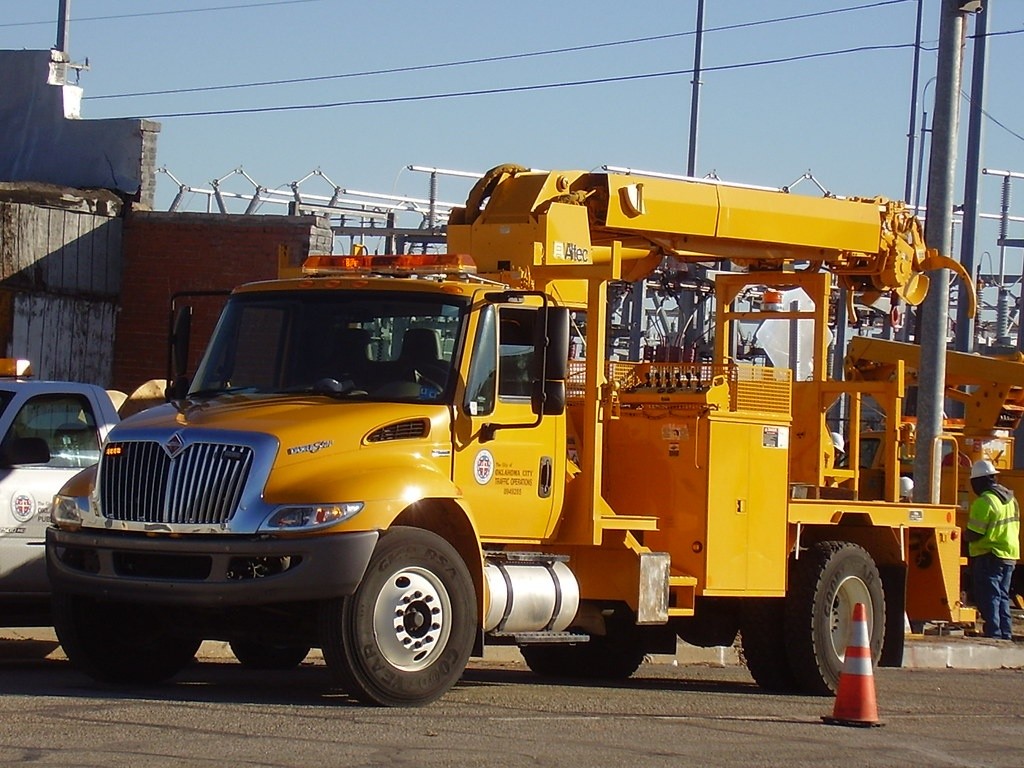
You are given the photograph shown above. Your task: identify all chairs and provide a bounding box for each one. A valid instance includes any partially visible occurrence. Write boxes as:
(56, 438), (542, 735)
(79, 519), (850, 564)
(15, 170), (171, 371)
(338, 329), (373, 362)
(395, 327), (443, 386)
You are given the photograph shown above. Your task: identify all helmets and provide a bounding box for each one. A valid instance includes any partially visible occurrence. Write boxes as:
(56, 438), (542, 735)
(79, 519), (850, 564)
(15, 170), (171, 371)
(831, 431), (845, 455)
(969, 459), (999, 479)
(898, 476), (916, 498)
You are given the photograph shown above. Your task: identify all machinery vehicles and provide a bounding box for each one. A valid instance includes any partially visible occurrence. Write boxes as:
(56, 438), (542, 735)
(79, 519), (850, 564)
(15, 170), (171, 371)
(43, 163), (979, 707)
(830, 334), (1023, 611)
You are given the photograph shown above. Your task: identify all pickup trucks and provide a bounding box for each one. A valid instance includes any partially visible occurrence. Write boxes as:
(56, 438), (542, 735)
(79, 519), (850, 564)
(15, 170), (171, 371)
(0, 357), (311, 668)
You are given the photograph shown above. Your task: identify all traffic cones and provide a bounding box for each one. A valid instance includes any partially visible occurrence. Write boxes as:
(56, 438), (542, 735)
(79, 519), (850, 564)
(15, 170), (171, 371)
(818, 603), (889, 729)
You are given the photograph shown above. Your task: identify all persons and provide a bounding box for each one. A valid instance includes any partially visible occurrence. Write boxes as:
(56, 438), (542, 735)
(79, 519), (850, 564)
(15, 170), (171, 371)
(832, 432), (845, 457)
(961, 459), (1020, 639)
(901, 477), (913, 498)
